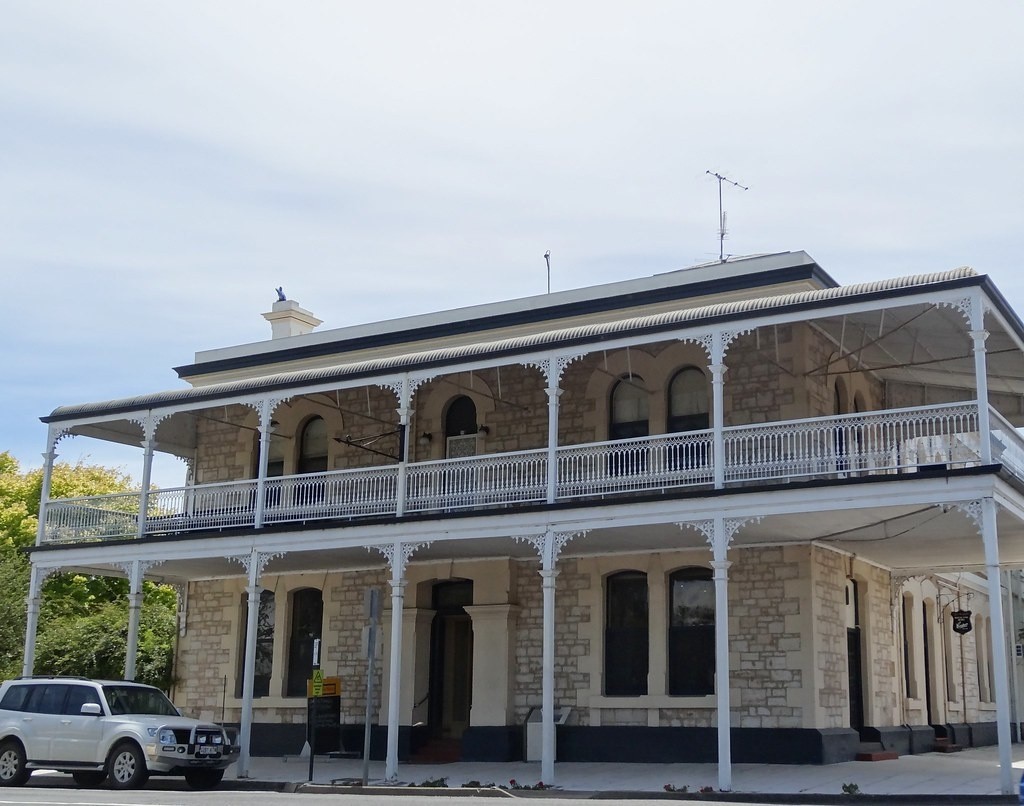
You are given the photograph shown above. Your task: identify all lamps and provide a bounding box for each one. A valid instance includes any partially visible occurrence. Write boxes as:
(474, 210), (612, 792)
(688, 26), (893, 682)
(420, 431), (433, 446)
(478, 424), (489, 440)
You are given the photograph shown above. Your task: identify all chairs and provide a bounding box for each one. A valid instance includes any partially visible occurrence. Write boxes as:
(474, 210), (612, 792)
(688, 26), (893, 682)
(114, 695), (130, 710)
(67, 692), (86, 715)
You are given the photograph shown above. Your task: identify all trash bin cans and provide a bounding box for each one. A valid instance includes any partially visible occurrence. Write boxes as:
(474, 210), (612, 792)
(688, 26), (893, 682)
(523, 707), (579, 764)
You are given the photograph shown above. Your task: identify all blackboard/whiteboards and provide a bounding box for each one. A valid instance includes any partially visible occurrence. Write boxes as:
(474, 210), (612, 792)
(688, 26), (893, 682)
(306, 694), (340, 751)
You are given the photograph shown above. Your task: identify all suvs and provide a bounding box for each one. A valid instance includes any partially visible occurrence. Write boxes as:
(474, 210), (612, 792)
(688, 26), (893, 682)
(0, 676), (241, 792)
